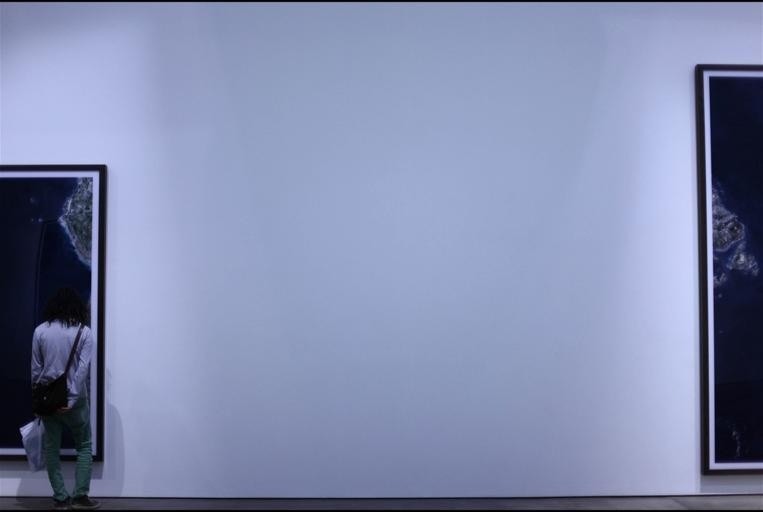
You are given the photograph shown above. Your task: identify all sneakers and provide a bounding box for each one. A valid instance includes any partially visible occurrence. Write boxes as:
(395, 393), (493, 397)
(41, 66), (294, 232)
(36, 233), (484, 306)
(70, 494), (101, 510)
(53, 496), (70, 511)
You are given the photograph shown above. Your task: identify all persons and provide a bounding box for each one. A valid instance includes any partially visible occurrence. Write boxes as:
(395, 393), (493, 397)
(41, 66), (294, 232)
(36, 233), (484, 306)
(29, 286), (102, 511)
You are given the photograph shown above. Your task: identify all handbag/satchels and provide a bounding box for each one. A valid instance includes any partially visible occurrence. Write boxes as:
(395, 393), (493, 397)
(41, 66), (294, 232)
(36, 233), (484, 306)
(19, 415), (47, 472)
(31, 373), (70, 426)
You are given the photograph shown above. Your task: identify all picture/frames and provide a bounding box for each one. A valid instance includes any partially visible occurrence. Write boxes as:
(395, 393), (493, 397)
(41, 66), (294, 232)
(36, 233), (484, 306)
(694, 62), (762, 472)
(0, 164), (105, 463)
